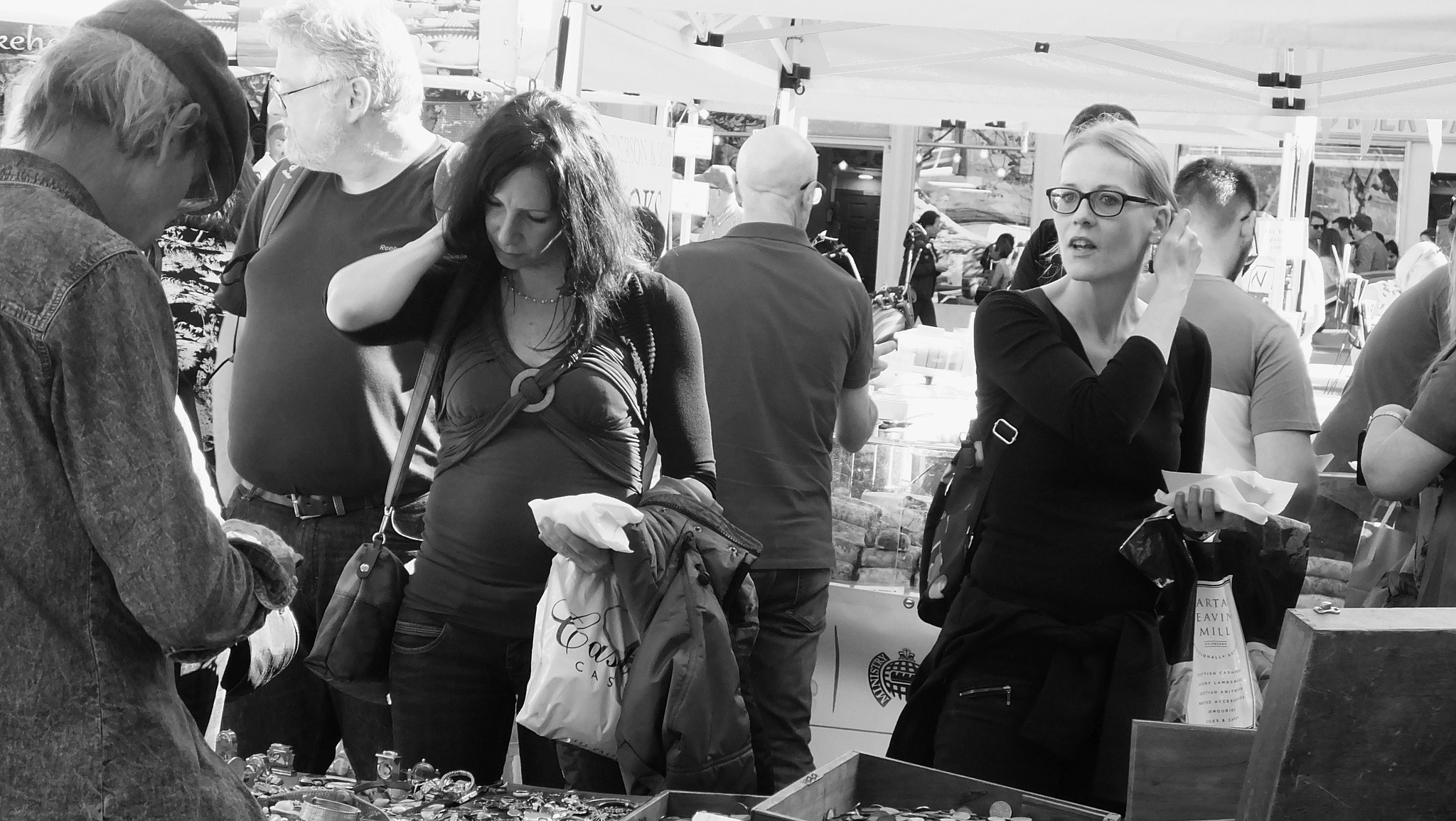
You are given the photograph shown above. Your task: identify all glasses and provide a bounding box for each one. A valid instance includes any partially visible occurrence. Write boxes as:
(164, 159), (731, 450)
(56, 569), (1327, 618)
(800, 179), (827, 205)
(268, 76), (336, 112)
(1310, 224), (1326, 230)
(175, 96), (219, 213)
(1046, 187), (1160, 218)
(1450, 194), (1456, 214)
(1240, 216), (1259, 267)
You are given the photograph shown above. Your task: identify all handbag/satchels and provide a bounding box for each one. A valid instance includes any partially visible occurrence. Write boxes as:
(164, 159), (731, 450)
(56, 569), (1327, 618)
(526, 492), (645, 556)
(1118, 504), (1313, 731)
(302, 538), (412, 707)
(1343, 499), (1416, 610)
(514, 550), (638, 765)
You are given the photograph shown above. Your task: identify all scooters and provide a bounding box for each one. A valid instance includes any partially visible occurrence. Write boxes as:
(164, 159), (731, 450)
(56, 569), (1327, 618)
(810, 221), (934, 343)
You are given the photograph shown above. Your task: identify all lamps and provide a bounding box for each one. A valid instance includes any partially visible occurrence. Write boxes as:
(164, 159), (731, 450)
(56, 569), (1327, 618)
(858, 162), (874, 179)
(831, 160), (848, 171)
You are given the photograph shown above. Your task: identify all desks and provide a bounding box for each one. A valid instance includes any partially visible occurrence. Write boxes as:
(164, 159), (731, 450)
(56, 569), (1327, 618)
(220, 758), (651, 821)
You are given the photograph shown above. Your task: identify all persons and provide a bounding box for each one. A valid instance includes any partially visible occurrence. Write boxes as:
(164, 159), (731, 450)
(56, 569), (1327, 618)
(218, 0), (477, 790)
(321, 87), (1456, 821)
(0, 1), (290, 821)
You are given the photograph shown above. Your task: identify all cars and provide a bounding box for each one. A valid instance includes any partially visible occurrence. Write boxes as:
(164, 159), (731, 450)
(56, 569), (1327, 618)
(909, 177), (1036, 306)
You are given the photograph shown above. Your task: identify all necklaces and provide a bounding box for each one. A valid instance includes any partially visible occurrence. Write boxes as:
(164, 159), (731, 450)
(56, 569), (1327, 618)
(500, 268), (566, 304)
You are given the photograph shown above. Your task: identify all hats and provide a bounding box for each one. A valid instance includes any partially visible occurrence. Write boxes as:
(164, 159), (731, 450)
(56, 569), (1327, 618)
(67, 0), (250, 221)
(694, 164), (737, 193)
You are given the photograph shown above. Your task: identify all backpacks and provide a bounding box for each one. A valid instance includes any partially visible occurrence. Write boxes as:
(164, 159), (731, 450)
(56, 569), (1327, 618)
(915, 288), (1195, 629)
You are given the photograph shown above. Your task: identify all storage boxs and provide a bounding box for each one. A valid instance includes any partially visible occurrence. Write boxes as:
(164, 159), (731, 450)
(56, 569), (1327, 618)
(751, 749), (1121, 821)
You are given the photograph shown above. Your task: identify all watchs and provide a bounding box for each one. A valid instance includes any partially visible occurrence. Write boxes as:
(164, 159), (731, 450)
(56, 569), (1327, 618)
(1365, 410), (1403, 432)
(1187, 530), (1215, 541)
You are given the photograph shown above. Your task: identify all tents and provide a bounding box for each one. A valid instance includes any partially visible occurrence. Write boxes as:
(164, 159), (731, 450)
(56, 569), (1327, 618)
(475, 2), (1455, 291)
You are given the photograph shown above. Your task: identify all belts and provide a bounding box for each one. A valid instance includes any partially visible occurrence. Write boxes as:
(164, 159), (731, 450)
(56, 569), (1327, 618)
(241, 474), (435, 520)
